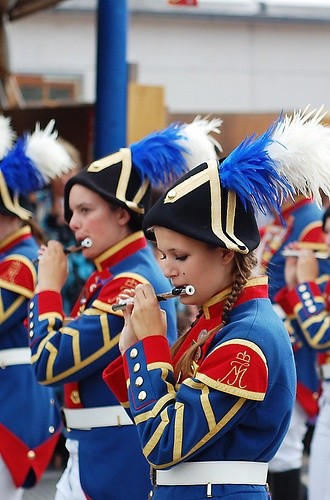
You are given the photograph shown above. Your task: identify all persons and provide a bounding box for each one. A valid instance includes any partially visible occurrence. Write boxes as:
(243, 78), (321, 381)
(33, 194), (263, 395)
(103, 104), (330, 500)
(0, 113), (330, 500)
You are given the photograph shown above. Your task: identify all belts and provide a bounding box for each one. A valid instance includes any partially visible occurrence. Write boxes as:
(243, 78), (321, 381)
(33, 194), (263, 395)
(150, 459), (268, 485)
(318, 364), (330, 381)
(0, 347), (33, 367)
(61, 405), (138, 431)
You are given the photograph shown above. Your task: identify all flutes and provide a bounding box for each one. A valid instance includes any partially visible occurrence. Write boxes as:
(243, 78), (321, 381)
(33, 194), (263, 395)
(33, 237), (93, 265)
(111, 284), (195, 312)
(281, 242), (330, 261)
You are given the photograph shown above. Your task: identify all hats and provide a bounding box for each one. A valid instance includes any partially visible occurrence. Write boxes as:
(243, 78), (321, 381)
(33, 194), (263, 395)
(0, 119), (75, 220)
(62, 114), (223, 227)
(141, 105), (330, 255)
